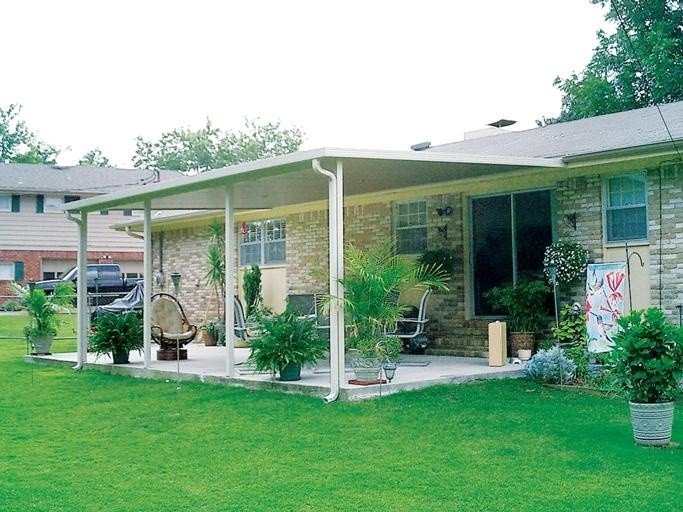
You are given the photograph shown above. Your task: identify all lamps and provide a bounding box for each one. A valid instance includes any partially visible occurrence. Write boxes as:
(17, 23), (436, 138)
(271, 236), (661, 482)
(432, 224), (447, 240)
(563, 212), (577, 231)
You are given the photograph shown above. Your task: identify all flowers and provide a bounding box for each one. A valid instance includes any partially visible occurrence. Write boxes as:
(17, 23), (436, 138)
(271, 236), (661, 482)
(543, 241), (591, 286)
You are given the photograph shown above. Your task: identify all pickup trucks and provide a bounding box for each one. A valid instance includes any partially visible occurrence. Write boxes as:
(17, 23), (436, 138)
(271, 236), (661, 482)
(27, 263), (144, 308)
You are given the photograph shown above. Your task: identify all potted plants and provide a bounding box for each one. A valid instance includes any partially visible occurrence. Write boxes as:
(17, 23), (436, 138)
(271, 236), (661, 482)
(605, 308), (683, 445)
(553, 303), (588, 348)
(202, 219), (263, 347)
(88, 310), (144, 366)
(317, 233), (449, 381)
(3, 281), (77, 355)
(486, 281), (552, 358)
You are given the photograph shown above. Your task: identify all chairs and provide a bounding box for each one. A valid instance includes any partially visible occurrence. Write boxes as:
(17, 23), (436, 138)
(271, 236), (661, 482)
(226, 289), (433, 384)
(149, 292), (197, 360)
